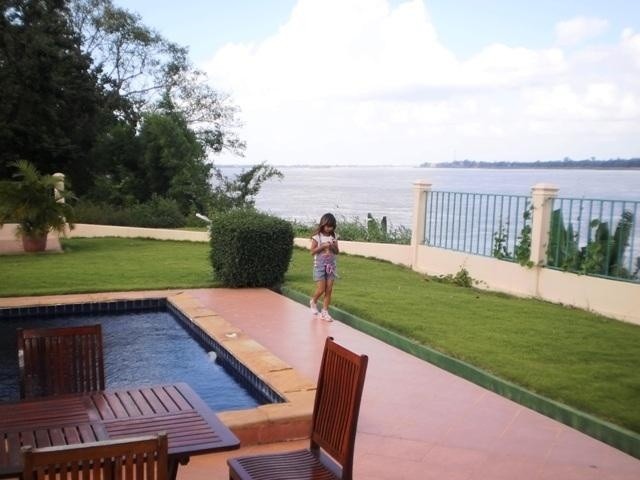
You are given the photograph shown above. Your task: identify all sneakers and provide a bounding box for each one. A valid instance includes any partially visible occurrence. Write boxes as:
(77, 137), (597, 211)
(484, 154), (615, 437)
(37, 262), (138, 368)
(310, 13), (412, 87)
(310, 298), (319, 315)
(321, 307), (333, 322)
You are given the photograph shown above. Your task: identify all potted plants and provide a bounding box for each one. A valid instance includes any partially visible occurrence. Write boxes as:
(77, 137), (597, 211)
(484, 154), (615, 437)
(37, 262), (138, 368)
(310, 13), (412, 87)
(0, 160), (80, 252)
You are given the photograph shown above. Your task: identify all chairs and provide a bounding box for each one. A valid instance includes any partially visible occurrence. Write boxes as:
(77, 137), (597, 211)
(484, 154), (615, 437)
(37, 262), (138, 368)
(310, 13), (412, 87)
(21, 430), (168, 480)
(227, 336), (368, 480)
(16, 324), (105, 399)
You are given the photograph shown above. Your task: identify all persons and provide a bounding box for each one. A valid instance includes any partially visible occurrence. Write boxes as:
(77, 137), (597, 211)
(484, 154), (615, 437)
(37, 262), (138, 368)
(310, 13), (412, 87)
(309, 212), (339, 323)
